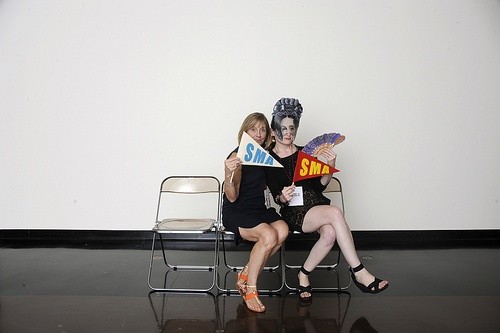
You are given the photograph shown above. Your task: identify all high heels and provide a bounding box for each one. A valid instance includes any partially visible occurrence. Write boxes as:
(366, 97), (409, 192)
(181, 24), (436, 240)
(296, 266), (312, 304)
(239, 285), (266, 312)
(349, 262), (389, 293)
(237, 266), (249, 292)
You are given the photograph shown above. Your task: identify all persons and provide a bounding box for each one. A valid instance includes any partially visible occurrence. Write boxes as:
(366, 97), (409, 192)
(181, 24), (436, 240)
(221, 111), (290, 312)
(266, 97), (390, 300)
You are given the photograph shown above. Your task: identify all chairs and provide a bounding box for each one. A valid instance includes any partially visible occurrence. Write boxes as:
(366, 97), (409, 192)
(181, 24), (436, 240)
(147, 176), (352, 291)
(148, 268), (351, 333)
